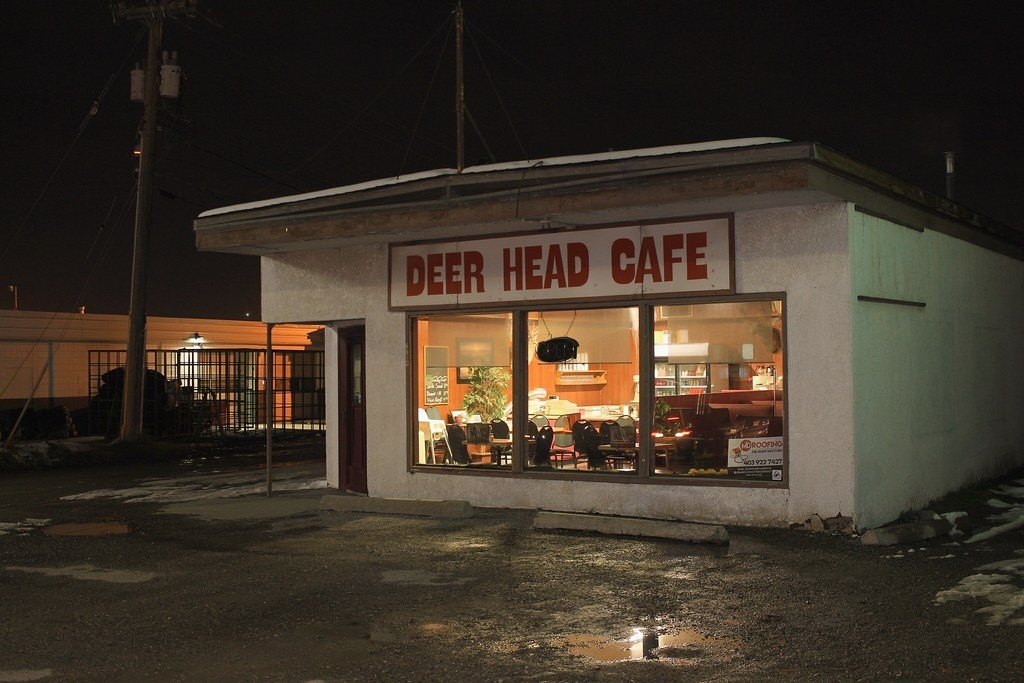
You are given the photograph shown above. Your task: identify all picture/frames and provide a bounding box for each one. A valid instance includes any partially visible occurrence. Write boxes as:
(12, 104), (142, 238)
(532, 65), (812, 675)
(455, 337), (494, 384)
(423, 345), (450, 406)
(659, 305), (693, 318)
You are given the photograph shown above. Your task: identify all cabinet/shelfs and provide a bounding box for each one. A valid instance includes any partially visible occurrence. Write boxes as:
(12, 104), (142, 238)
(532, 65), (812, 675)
(752, 375), (779, 391)
(556, 370), (608, 386)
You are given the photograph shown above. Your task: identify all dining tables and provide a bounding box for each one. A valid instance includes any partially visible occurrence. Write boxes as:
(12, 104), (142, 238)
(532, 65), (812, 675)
(461, 438), (536, 466)
(536, 426), (573, 434)
(598, 442), (658, 470)
(446, 423), (492, 433)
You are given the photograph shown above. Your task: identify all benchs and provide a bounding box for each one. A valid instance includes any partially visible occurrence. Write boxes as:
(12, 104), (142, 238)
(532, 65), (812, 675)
(669, 400), (783, 468)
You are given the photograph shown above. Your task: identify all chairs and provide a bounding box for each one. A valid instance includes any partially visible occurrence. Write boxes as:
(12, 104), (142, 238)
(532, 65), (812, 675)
(418, 407), (640, 470)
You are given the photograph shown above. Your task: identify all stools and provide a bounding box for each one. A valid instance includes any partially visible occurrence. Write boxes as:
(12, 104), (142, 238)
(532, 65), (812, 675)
(655, 443), (678, 469)
